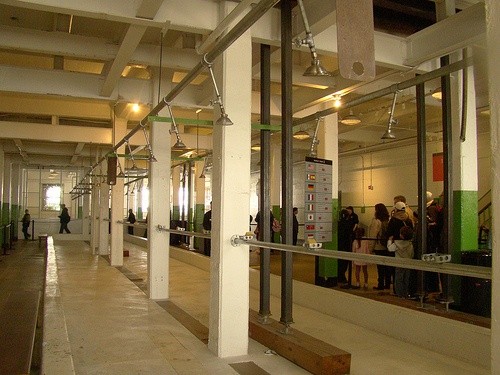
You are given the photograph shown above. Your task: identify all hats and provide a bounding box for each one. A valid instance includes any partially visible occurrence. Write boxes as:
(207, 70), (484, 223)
(61, 204), (66, 206)
(394, 202), (405, 210)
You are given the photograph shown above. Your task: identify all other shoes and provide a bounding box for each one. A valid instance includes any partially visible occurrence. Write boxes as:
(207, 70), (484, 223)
(376, 287), (384, 290)
(357, 282), (360, 286)
(364, 284), (368, 288)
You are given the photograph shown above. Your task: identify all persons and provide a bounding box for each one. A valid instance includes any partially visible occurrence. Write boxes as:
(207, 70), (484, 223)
(56, 204), (71, 235)
(202, 202), (212, 257)
(21, 208), (32, 241)
(254, 205), (360, 251)
(125, 207), (136, 235)
(328, 191), (447, 298)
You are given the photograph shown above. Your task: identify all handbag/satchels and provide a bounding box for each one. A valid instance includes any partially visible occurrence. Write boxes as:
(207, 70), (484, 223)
(66, 215), (70, 224)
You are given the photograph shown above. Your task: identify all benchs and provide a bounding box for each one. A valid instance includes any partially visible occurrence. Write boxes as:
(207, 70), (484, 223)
(38, 233), (48, 247)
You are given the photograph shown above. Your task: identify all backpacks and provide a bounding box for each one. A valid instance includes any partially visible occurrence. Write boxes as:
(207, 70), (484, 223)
(271, 218), (281, 232)
